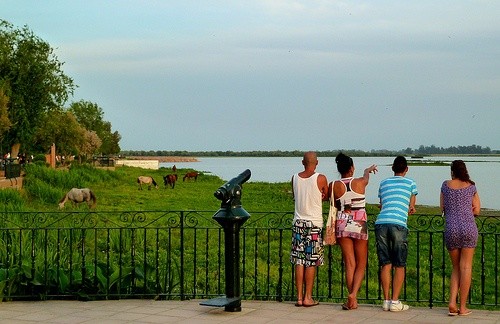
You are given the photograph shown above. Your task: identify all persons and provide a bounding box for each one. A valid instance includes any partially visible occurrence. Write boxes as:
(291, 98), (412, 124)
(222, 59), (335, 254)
(4, 152), (26, 164)
(328, 152), (378, 309)
(291, 151), (328, 306)
(440, 160), (481, 316)
(374, 156), (418, 311)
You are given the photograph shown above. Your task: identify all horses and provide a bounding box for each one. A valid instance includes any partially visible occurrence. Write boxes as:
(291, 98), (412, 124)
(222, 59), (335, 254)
(57, 186), (97, 209)
(163, 173), (177, 188)
(137, 176), (158, 189)
(182, 171), (199, 182)
(172, 165), (176, 171)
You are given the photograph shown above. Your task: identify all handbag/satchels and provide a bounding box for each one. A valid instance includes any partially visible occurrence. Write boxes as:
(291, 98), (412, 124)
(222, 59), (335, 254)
(322, 182), (338, 246)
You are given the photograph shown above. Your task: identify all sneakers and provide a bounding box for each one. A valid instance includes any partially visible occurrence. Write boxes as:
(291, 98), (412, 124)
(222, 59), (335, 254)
(389, 303), (409, 311)
(383, 303), (391, 311)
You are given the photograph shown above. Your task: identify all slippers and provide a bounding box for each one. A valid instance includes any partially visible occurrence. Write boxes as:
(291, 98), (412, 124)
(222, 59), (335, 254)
(459, 310), (473, 315)
(303, 299), (319, 307)
(448, 311), (459, 315)
(295, 301), (302, 306)
(342, 303), (351, 310)
